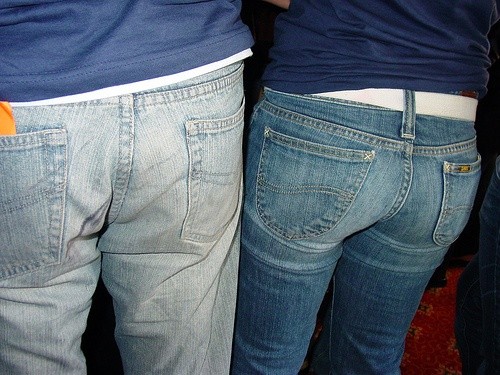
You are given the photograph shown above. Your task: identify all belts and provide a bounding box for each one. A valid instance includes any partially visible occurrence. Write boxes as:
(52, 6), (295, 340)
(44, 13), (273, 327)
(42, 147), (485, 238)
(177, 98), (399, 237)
(311, 88), (477, 122)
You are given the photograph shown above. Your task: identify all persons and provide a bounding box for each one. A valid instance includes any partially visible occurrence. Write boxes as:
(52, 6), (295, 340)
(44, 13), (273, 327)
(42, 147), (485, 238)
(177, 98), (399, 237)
(0, 0), (253, 375)
(230, 0), (499, 375)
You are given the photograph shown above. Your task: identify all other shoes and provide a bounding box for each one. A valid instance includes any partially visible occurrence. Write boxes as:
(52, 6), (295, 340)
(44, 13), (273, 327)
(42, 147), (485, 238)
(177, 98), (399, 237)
(427, 272), (447, 287)
